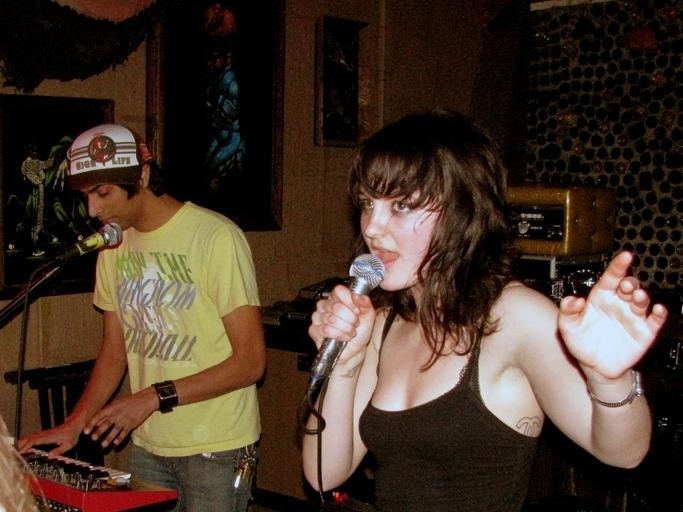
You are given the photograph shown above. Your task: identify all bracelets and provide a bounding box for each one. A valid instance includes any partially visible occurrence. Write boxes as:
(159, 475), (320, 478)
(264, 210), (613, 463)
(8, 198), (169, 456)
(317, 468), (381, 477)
(585, 368), (644, 408)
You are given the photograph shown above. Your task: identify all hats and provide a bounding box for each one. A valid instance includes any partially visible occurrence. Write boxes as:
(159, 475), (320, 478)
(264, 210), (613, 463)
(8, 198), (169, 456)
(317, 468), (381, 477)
(65, 124), (156, 191)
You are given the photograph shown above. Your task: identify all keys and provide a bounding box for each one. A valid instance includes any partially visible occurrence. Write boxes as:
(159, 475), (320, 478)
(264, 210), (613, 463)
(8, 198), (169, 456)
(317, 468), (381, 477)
(232, 453), (257, 491)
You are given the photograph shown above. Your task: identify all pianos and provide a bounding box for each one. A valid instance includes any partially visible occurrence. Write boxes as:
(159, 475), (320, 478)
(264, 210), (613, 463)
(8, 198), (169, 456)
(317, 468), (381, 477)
(12, 441), (179, 511)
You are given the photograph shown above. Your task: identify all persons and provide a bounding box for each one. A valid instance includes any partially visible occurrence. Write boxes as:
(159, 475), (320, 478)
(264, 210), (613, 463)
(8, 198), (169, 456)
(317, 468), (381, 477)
(14, 122), (270, 512)
(296, 110), (672, 512)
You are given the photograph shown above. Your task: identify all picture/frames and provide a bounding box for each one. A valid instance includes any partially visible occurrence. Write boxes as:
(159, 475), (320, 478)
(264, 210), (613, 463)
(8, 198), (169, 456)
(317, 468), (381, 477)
(146, 2), (290, 233)
(0, 91), (117, 299)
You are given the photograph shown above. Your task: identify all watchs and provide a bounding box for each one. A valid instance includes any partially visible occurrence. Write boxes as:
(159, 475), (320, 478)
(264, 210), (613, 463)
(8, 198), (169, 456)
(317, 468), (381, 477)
(151, 379), (179, 414)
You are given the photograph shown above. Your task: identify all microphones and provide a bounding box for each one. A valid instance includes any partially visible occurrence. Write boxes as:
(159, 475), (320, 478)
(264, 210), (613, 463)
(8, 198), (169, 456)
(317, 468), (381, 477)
(42, 222), (122, 268)
(307, 254), (386, 399)
